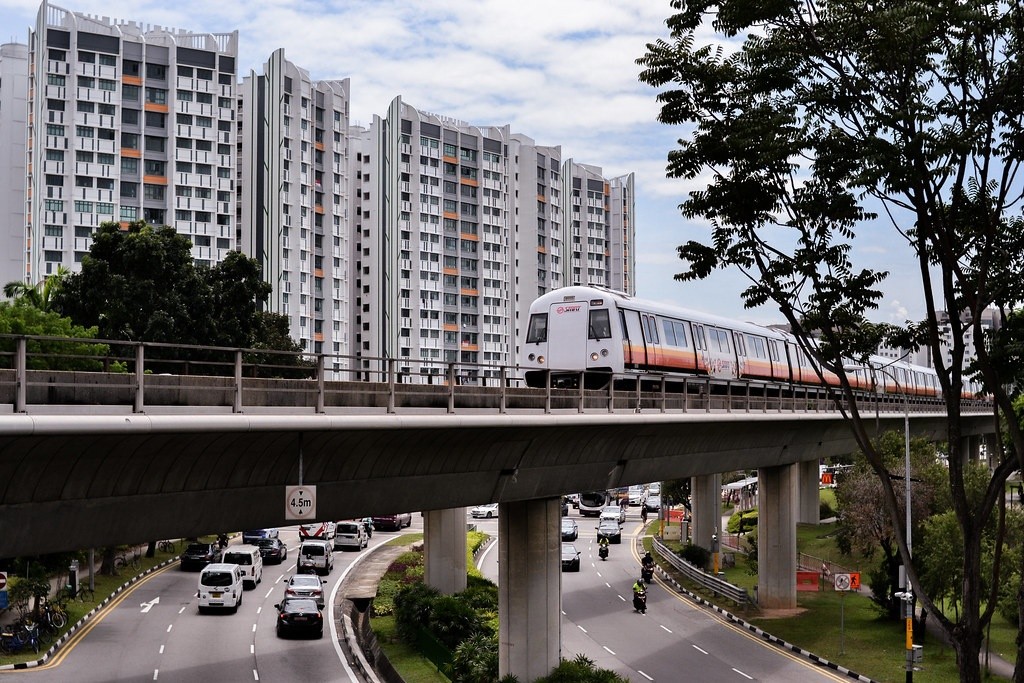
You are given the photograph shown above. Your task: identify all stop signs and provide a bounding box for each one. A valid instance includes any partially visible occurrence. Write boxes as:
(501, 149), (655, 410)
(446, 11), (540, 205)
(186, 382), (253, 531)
(0, 573), (6, 589)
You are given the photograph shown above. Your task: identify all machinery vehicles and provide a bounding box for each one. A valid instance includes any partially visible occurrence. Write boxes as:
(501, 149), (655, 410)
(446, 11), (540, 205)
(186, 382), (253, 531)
(577, 492), (610, 517)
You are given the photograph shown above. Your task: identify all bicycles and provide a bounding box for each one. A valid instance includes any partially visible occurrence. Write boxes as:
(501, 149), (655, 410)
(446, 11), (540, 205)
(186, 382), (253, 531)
(56, 583), (95, 602)
(115, 551), (142, 571)
(158, 539), (175, 554)
(0, 599), (69, 655)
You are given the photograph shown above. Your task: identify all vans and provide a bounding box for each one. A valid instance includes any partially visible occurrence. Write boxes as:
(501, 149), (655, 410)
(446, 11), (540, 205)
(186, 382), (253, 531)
(198, 562), (244, 614)
(299, 522), (336, 543)
(296, 539), (334, 575)
(334, 521), (368, 551)
(221, 544), (263, 590)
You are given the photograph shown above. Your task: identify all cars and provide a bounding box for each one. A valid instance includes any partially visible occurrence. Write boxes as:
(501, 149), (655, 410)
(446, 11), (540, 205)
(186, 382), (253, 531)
(594, 520), (624, 544)
(599, 505), (626, 524)
(628, 490), (647, 506)
(647, 483), (661, 496)
(273, 599), (326, 638)
(643, 496), (660, 512)
(373, 512), (412, 531)
(181, 542), (223, 571)
(242, 527), (279, 543)
(470, 503), (498, 518)
(562, 544), (581, 572)
(561, 519), (579, 541)
(283, 573), (327, 606)
(253, 538), (288, 564)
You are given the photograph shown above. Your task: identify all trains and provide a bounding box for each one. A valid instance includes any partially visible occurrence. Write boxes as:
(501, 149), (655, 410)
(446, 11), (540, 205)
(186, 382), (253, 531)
(520, 283), (995, 407)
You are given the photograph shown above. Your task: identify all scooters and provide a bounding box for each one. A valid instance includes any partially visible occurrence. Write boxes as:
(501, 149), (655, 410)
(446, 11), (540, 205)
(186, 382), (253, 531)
(632, 586), (649, 613)
(598, 544), (609, 561)
(640, 562), (658, 585)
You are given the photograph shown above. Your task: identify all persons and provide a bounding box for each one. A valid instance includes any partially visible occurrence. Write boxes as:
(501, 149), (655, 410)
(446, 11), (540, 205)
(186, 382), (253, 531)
(633, 579), (646, 604)
(607, 493), (619, 506)
(641, 506), (647, 527)
(599, 535), (609, 553)
(641, 551), (656, 579)
(738, 514), (745, 536)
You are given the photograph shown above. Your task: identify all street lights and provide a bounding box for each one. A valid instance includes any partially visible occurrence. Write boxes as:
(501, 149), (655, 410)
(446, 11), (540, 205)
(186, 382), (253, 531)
(845, 365), (913, 683)
